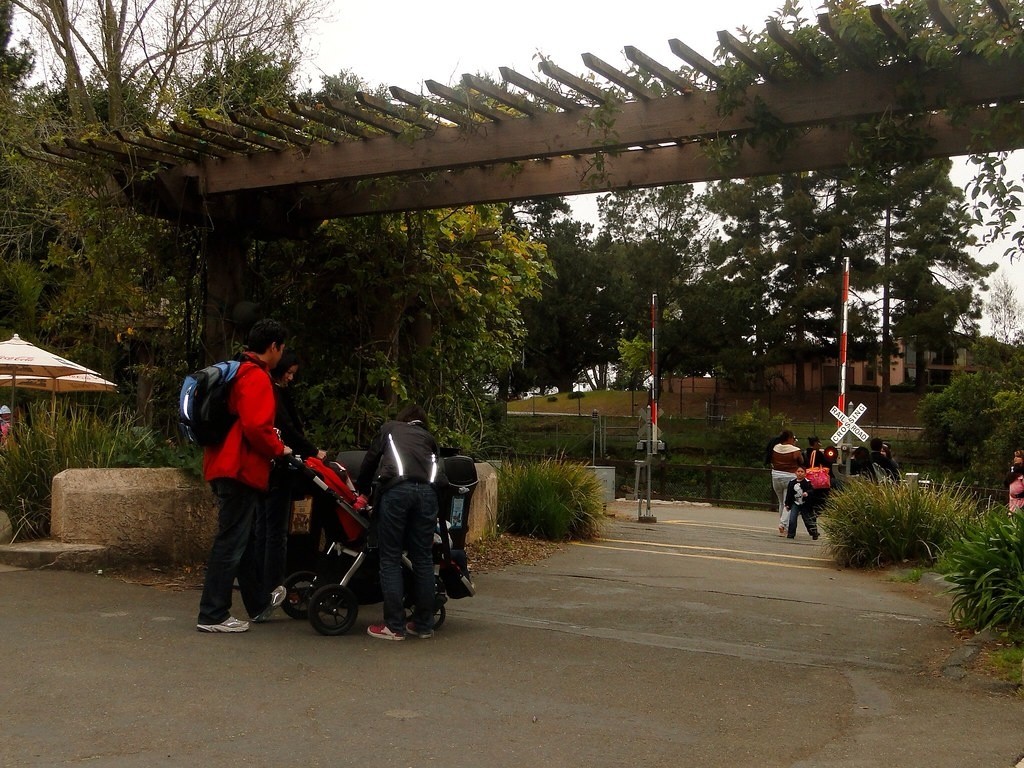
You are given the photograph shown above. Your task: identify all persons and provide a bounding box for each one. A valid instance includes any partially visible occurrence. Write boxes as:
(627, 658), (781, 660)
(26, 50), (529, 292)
(357, 402), (442, 645)
(770, 428), (903, 550)
(0, 403), (16, 454)
(195, 319), (355, 636)
(1005, 448), (1024, 519)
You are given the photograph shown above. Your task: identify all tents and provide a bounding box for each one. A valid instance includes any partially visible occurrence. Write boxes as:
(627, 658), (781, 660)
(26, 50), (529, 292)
(0, 332), (120, 400)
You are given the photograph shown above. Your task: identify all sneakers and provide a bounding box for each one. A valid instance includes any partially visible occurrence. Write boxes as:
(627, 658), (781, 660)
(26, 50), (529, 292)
(252, 586), (286, 622)
(406, 622), (431, 638)
(367, 623), (405, 641)
(197, 616), (250, 633)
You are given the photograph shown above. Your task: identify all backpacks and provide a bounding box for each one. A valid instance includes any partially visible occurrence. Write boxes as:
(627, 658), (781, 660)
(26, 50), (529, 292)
(179, 352), (264, 447)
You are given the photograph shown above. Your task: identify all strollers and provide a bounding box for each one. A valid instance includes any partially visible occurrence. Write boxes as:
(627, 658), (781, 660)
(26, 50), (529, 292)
(274, 454), (475, 634)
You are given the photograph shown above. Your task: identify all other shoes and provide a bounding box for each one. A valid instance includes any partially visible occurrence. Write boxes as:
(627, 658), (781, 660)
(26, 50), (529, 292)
(810, 533), (820, 536)
(779, 527), (786, 537)
(787, 535), (794, 539)
(813, 535), (818, 540)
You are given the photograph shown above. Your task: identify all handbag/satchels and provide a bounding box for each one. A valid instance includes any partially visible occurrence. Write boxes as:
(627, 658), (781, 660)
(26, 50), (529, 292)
(804, 450), (830, 489)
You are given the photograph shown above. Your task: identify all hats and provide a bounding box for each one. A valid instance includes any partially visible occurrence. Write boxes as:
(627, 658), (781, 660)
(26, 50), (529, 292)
(0, 405), (11, 414)
